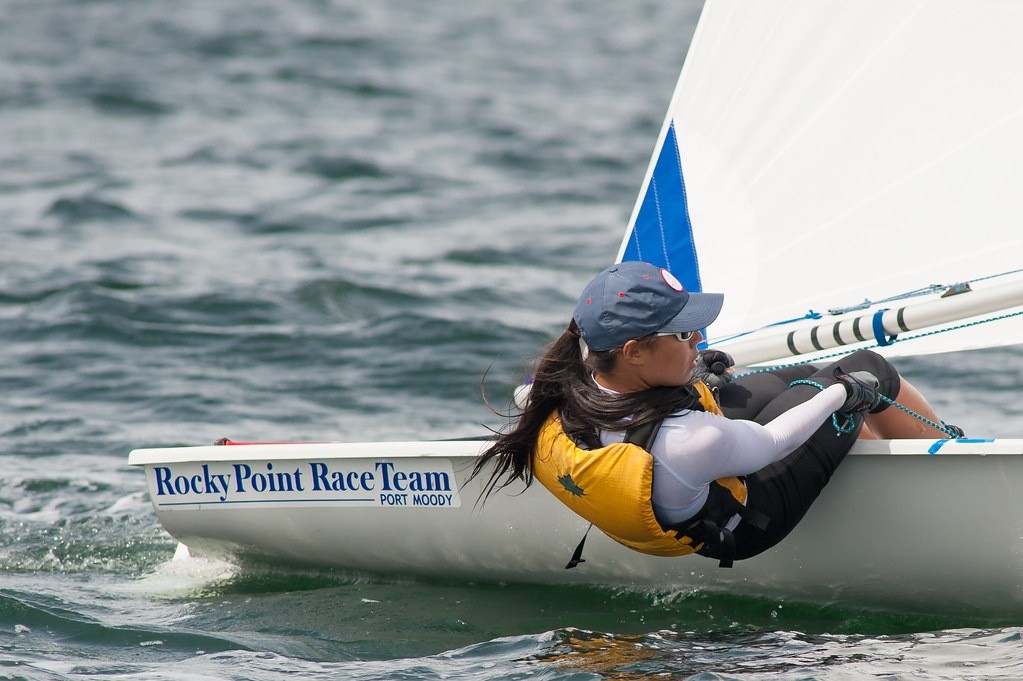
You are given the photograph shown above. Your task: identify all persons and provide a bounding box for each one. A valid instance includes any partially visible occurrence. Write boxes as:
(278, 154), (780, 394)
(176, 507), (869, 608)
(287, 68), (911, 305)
(459, 261), (963, 568)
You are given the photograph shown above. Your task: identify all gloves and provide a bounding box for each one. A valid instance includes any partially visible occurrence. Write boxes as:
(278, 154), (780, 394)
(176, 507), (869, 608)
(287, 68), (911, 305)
(835, 370), (880, 415)
(690, 348), (735, 389)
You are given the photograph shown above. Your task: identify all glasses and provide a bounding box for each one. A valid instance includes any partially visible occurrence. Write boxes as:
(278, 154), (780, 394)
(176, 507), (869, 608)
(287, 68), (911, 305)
(657, 330), (694, 342)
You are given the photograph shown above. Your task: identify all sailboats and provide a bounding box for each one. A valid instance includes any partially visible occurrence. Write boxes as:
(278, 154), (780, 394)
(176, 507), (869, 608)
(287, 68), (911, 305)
(127, 0), (1023, 615)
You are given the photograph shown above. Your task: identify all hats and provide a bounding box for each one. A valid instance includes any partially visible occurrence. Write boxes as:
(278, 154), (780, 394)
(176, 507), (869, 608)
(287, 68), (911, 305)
(573, 260), (725, 351)
